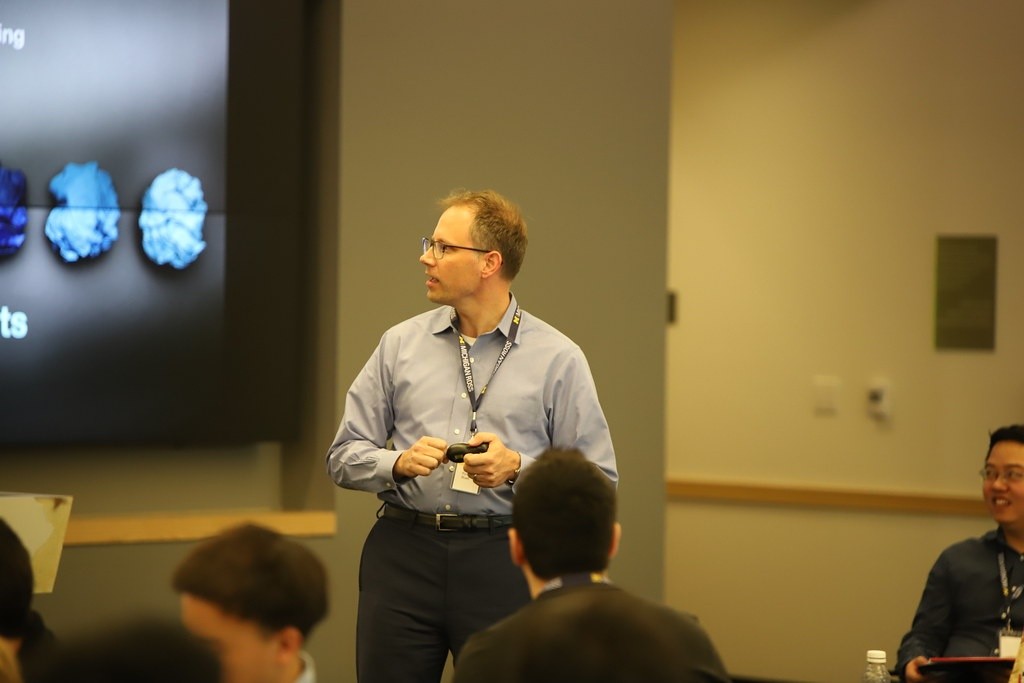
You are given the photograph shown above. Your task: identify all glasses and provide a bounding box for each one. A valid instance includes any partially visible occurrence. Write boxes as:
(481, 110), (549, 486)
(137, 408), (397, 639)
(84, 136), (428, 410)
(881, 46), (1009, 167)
(421, 237), (504, 267)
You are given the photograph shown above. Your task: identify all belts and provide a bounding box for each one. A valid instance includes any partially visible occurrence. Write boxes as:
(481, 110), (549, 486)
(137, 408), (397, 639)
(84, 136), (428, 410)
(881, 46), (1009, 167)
(383, 502), (516, 533)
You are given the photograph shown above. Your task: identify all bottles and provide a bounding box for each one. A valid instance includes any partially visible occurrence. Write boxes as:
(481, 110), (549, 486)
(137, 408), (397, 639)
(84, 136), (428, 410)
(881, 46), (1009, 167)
(861, 649), (890, 683)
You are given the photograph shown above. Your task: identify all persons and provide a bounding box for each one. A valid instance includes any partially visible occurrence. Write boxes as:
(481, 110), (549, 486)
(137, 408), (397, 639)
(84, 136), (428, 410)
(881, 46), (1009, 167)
(327, 192), (619, 682)
(889, 423), (1024, 683)
(449, 446), (727, 683)
(174, 522), (332, 681)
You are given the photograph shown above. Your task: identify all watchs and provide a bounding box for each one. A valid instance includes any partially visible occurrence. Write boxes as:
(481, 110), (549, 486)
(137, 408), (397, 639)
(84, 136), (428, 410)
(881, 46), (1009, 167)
(506, 467), (521, 484)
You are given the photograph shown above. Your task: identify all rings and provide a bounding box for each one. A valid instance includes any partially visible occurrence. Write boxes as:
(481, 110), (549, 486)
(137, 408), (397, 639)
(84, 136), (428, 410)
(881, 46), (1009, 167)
(473, 473), (478, 482)
